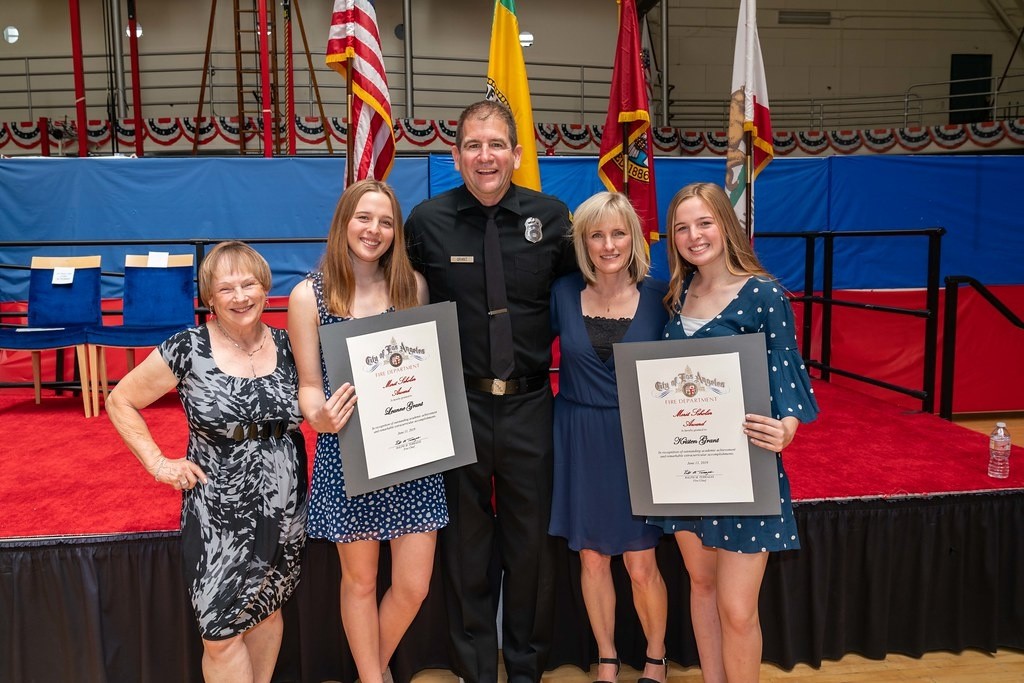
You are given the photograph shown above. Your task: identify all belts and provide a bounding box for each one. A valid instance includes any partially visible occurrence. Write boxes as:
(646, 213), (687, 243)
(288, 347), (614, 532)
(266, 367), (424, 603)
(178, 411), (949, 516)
(464, 373), (548, 397)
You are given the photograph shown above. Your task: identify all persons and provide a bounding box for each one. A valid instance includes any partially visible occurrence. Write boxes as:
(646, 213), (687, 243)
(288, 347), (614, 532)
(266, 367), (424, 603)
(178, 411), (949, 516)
(547, 192), (668, 683)
(104, 240), (305, 683)
(646, 182), (821, 683)
(286, 179), (450, 683)
(405, 101), (574, 683)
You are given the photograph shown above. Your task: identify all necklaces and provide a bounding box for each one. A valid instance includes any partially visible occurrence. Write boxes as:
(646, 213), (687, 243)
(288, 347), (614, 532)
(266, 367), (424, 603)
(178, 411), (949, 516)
(690, 265), (735, 299)
(595, 278), (629, 314)
(216, 317), (266, 378)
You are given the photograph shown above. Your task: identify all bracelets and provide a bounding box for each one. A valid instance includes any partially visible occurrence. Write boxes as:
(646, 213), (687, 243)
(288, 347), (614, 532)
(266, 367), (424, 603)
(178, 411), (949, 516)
(155, 457), (165, 482)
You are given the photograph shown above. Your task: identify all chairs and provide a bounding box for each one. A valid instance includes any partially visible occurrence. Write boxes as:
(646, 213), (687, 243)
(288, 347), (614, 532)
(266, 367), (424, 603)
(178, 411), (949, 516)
(1, 253), (107, 419)
(85, 252), (196, 419)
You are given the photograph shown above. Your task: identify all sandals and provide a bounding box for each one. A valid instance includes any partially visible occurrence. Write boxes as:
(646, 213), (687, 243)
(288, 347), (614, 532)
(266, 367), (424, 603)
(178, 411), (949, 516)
(638, 655), (668, 683)
(592, 657), (621, 683)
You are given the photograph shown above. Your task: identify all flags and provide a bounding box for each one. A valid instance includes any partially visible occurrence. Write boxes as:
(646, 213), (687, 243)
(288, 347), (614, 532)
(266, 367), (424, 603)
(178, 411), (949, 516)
(326, 0), (396, 192)
(724, 0), (774, 249)
(598, 0), (660, 265)
(486, 0), (541, 193)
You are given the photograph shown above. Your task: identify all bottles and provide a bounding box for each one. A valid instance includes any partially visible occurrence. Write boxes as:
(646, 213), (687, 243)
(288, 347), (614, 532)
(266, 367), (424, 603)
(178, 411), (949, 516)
(987, 422), (1011, 478)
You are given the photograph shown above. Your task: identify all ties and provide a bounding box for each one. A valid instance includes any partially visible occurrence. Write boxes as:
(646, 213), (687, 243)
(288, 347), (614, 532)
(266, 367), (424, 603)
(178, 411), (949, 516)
(484, 207), (516, 381)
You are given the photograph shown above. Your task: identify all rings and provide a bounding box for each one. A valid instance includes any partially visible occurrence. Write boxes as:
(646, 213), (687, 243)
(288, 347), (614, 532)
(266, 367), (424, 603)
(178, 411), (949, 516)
(179, 481), (187, 485)
